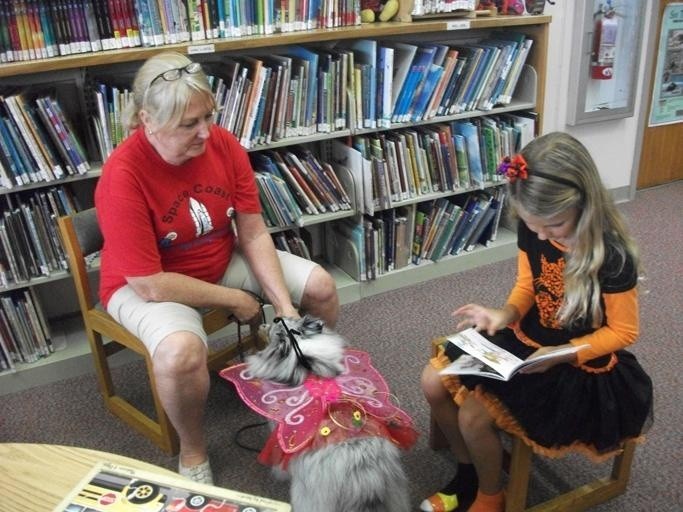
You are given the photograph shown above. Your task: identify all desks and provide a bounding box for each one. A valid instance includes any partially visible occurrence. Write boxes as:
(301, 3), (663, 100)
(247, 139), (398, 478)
(0, 440), (192, 512)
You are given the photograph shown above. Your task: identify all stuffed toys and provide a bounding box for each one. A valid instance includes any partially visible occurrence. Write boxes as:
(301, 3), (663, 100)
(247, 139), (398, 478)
(361, 0), (399, 23)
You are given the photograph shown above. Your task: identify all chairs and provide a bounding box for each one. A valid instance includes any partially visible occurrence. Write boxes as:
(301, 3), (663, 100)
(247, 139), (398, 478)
(58, 208), (264, 457)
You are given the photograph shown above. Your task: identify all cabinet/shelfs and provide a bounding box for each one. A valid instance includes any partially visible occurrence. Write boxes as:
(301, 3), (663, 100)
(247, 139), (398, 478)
(0, 15), (552, 396)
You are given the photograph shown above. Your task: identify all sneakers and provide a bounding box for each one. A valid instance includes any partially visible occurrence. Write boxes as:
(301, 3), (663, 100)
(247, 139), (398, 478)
(178, 454), (213, 486)
(419, 462), (479, 512)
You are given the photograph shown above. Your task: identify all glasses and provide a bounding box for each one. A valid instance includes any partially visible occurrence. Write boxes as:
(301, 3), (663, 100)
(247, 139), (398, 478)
(143, 62), (202, 107)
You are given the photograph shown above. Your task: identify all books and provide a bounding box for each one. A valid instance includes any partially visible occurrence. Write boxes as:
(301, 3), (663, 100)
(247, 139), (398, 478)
(275, 190), (505, 280)
(205, 33), (533, 148)
(436, 327), (591, 382)
(251, 111), (539, 228)
(1, 184), (89, 372)
(0, 80), (133, 188)
(0, 1), (361, 61)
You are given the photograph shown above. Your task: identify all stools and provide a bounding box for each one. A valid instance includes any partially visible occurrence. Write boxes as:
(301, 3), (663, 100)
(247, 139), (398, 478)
(429, 337), (637, 512)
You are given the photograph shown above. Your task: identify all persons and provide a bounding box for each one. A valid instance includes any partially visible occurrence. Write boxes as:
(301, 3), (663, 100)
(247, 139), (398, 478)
(93, 52), (338, 487)
(417, 130), (653, 512)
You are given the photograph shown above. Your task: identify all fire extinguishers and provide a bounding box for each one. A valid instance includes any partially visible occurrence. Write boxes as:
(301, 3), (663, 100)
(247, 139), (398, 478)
(590, 0), (628, 79)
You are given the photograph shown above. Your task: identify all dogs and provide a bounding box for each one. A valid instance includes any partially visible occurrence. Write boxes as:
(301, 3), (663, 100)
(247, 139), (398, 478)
(242, 310), (412, 512)
